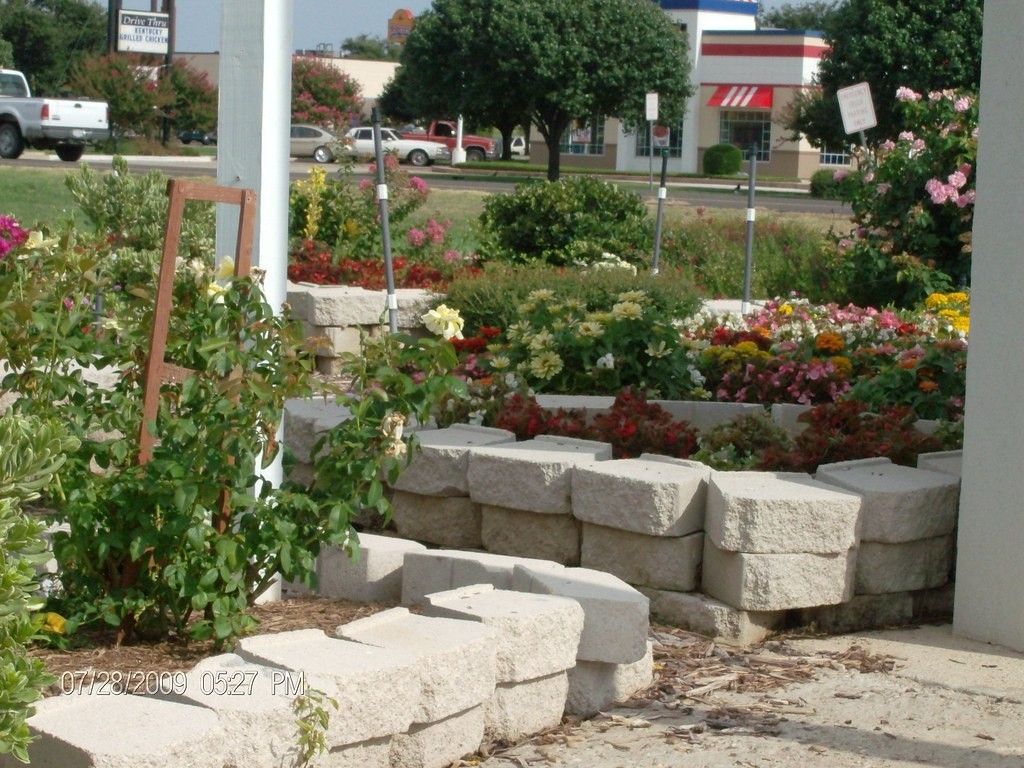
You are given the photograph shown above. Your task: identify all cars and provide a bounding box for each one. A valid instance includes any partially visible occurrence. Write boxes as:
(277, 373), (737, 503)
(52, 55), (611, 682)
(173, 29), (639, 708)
(343, 127), (447, 166)
(176, 130), (217, 145)
(290, 123), (358, 163)
(511, 136), (531, 155)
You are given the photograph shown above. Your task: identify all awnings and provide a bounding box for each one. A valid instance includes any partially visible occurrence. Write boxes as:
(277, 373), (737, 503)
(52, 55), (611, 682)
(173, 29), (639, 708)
(707, 84), (772, 110)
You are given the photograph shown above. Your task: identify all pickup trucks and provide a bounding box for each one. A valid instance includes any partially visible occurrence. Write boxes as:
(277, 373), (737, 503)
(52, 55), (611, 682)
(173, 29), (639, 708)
(0, 69), (110, 162)
(401, 120), (499, 161)
